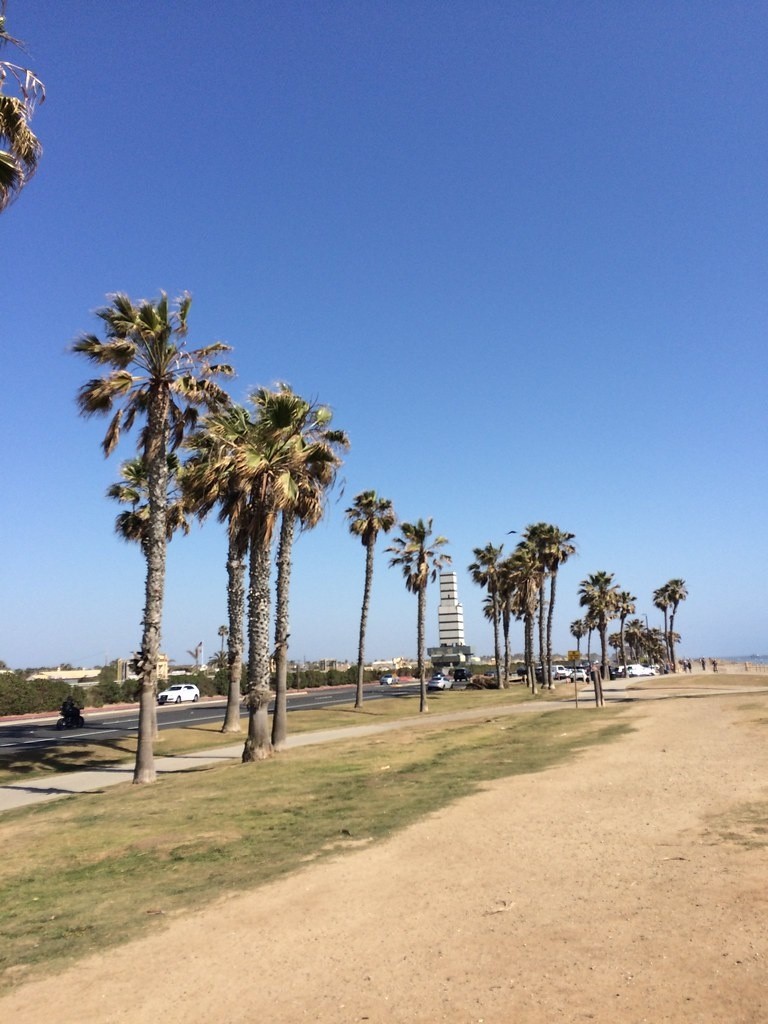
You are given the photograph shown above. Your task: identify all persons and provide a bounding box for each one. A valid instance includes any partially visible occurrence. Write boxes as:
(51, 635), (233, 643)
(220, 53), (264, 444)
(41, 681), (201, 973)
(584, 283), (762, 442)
(712, 659), (718, 673)
(701, 657), (706, 671)
(61, 695), (75, 724)
(682, 662), (691, 672)
(666, 661), (675, 673)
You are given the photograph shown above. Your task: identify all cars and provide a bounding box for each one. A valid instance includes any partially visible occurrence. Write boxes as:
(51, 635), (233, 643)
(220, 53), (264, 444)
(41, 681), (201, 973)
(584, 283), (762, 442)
(380, 674), (400, 684)
(158, 683), (200, 705)
(432, 673), (446, 677)
(609, 663), (659, 677)
(536, 665), (572, 679)
(485, 671), (498, 677)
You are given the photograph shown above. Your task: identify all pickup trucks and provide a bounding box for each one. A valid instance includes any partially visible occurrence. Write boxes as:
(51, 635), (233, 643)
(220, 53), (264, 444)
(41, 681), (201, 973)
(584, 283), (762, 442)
(570, 670), (588, 681)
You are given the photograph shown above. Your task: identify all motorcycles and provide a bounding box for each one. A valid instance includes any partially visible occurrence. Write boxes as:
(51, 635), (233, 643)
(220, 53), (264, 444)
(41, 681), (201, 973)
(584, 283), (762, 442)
(56, 707), (85, 731)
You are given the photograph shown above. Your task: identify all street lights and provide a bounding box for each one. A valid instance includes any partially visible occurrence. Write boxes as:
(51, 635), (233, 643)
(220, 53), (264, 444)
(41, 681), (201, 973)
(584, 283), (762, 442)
(641, 614), (652, 665)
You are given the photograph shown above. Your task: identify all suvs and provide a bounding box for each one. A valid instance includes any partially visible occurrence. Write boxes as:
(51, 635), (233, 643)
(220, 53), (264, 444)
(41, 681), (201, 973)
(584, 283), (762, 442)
(454, 668), (474, 681)
(426, 677), (454, 690)
